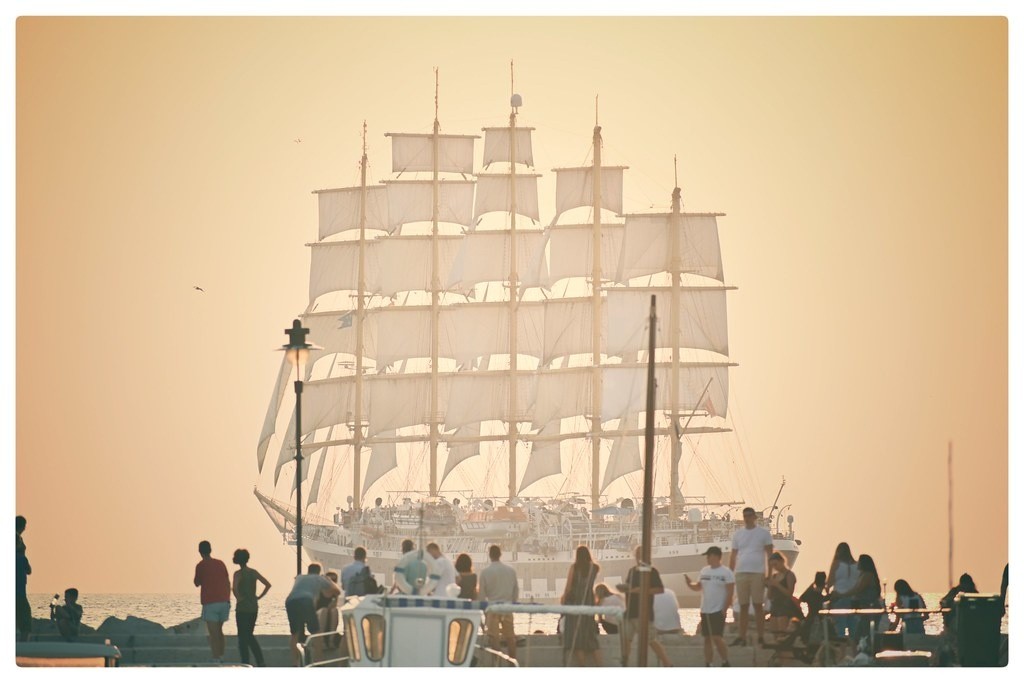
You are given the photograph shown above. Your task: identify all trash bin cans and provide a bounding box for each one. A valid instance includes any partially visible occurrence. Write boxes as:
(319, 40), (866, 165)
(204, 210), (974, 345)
(954, 591), (1001, 667)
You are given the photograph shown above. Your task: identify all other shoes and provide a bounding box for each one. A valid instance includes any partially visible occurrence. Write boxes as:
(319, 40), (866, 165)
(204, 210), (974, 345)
(758, 637), (765, 645)
(722, 661), (730, 667)
(728, 638), (746, 647)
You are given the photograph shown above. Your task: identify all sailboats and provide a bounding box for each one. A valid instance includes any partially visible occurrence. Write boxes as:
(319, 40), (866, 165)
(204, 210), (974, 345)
(254, 59), (799, 606)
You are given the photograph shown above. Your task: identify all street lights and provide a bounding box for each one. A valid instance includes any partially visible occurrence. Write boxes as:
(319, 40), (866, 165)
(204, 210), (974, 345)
(272, 318), (325, 576)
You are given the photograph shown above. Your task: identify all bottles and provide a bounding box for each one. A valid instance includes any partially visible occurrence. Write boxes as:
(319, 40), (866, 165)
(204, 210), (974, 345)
(50, 594), (59, 607)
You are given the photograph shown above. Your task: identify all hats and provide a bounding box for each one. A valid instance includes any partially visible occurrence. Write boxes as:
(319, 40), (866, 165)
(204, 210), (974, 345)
(701, 547), (722, 556)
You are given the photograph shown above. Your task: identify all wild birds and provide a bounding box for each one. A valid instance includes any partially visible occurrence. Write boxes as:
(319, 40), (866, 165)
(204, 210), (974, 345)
(193, 284), (205, 294)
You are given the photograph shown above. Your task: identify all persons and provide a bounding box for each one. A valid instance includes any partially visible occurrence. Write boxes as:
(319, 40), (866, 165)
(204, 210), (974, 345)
(595, 585), (626, 633)
(285, 564), (341, 667)
(50, 588), (83, 642)
(652, 589), (681, 634)
(562, 546), (605, 666)
(999, 564), (1009, 658)
(232, 549), (272, 666)
(478, 545), (518, 667)
(940, 574), (978, 635)
(687, 546), (735, 667)
(890, 580), (929, 634)
(194, 540), (231, 663)
(621, 546), (673, 667)
(16, 515), (32, 640)
(729, 508), (775, 647)
(342, 539), (478, 601)
(308, 571), (338, 650)
(762, 543), (880, 663)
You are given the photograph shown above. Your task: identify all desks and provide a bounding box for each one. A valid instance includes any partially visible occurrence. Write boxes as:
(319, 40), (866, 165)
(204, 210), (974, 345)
(15, 641), (121, 667)
(820, 607), (952, 667)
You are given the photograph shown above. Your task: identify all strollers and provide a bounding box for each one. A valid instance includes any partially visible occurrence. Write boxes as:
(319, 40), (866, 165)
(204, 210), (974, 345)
(763, 592), (846, 667)
